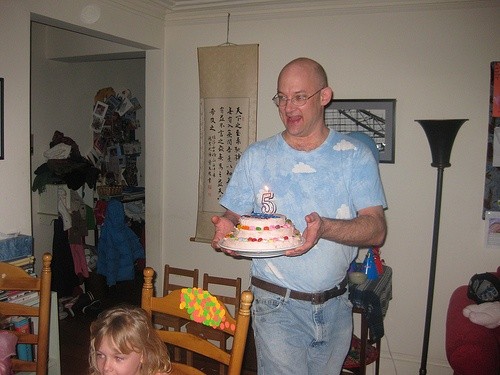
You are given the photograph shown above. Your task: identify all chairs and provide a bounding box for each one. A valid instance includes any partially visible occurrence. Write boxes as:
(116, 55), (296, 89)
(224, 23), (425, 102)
(141, 266), (255, 375)
(154, 264), (199, 362)
(0, 252), (53, 375)
(185, 274), (242, 375)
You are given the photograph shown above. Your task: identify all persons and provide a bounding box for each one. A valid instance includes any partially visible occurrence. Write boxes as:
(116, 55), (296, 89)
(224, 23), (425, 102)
(86, 302), (173, 375)
(210, 58), (387, 375)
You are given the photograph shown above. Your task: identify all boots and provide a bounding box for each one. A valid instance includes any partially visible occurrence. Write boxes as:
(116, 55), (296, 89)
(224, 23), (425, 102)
(67, 291), (94, 317)
(83, 300), (105, 315)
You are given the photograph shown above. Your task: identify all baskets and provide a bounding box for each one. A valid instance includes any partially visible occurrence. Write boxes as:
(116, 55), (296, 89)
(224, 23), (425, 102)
(97, 185), (124, 196)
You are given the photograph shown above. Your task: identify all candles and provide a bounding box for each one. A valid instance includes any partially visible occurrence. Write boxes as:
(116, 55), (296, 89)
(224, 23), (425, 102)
(260, 185), (276, 215)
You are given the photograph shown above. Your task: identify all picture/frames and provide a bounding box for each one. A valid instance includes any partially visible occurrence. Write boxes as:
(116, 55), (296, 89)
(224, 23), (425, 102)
(327, 98), (397, 164)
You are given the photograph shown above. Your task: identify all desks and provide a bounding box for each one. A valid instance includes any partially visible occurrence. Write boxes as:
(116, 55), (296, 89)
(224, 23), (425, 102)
(342, 265), (393, 375)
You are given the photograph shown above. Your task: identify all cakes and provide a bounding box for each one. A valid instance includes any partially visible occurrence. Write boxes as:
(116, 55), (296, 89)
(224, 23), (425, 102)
(222, 212), (304, 251)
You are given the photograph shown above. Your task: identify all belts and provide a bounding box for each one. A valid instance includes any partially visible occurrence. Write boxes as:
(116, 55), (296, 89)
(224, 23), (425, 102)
(250, 276), (349, 304)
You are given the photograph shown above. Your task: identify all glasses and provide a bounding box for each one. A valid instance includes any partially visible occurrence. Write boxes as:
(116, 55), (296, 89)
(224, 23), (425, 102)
(272, 88), (323, 106)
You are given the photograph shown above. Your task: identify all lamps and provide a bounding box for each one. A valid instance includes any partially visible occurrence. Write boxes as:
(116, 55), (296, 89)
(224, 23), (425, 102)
(414, 118), (471, 375)
